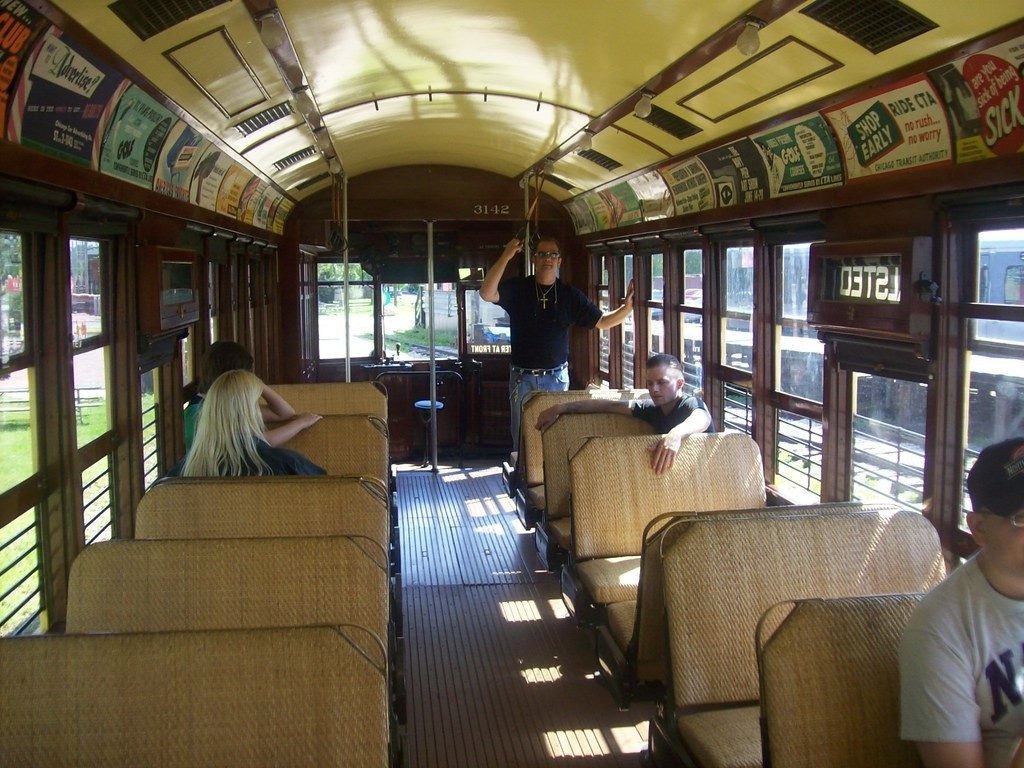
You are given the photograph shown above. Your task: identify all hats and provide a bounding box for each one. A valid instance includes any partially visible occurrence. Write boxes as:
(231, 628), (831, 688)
(966, 437), (1024, 509)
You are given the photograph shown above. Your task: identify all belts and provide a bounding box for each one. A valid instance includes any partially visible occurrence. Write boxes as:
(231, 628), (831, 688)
(512, 364), (567, 375)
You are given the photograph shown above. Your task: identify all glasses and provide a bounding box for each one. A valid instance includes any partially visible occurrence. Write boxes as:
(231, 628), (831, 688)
(534, 250), (561, 261)
(986, 509), (1024, 528)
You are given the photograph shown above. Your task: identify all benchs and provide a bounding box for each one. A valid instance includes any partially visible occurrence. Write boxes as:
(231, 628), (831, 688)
(0, 380), (412, 768)
(500, 387), (947, 767)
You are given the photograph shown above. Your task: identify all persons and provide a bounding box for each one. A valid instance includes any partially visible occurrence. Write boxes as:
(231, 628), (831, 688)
(166, 369), (329, 477)
(480, 238), (635, 452)
(184, 342), (324, 454)
(535, 354), (715, 474)
(899, 437), (1024, 768)
(763, 145), (785, 193)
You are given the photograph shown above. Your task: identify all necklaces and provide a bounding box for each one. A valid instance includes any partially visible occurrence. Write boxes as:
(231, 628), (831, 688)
(536, 283), (554, 309)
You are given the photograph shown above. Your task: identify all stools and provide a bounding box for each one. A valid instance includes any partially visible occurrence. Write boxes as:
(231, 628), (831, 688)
(415, 400), (444, 467)
